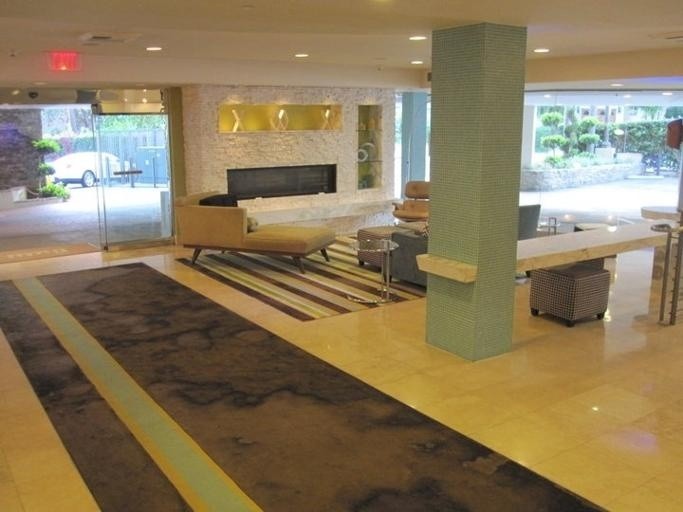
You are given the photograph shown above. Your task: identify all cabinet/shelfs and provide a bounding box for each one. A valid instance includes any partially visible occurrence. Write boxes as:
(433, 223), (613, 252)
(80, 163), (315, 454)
(355, 128), (381, 163)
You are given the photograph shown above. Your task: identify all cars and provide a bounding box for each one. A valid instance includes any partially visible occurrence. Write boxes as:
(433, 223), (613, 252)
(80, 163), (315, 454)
(46, 151), (142, 188)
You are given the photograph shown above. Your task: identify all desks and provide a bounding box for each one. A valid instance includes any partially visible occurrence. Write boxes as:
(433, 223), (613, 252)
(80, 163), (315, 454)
(536, 215), (561, 236)
(349, 239), (401, 304)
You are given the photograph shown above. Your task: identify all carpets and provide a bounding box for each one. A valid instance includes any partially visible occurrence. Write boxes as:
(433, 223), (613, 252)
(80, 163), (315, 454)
(173, 230), (427, 323)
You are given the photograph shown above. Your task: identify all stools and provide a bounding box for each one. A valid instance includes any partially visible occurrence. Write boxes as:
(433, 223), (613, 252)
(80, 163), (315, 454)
(357, 223), (405, 272)
(527, 265), (610, 326)
(574, 221), (618, 282)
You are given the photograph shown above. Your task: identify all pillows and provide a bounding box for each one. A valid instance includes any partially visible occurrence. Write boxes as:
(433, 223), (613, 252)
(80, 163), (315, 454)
(199, 194), (239, 207)
(400, 221), (429, 237)
(246, 217), (259, 232)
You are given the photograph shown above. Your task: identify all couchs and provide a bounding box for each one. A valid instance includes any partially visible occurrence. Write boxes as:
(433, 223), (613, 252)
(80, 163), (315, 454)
(381, 202), (542, 290)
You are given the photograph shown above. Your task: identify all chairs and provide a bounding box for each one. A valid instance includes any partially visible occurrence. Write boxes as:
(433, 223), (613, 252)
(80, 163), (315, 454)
(391, 179), (430, 228)
(173, 190), (336, 275)
